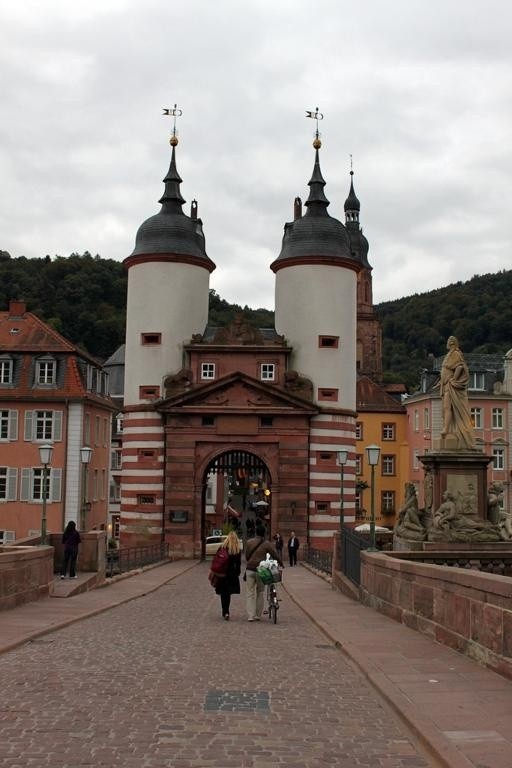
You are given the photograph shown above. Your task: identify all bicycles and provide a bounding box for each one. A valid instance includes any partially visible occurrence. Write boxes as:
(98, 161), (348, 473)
(258, 567), (285, 623)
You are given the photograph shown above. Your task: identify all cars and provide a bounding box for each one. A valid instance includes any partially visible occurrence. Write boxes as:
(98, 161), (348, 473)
(205, 534), (245, 555)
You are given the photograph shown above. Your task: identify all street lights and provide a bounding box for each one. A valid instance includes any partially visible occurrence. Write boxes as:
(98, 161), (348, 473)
(37, 443), (54, 544)
(334, 446), (349, 530)
(80, 444), (93, 530)
(364, 440), (382, 551)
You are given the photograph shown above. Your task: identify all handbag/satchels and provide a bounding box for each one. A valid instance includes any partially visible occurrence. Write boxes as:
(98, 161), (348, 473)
(210, 548), (228, 574)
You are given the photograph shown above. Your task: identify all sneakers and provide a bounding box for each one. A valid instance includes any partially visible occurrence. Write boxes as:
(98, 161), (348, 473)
(225, 614), (229, 619)
(248, 616), (261, 621)
(60, 575), (77, 579)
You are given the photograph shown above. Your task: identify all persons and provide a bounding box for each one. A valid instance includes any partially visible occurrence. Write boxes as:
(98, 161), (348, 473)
(399, 481), (427, 534)
(433, 489), (504, 535)
(60, 521), (82, 579)
(487, 479), (512, 542)
(207, 529), (242, 621)
(272, 531), (283, 562)
(243, 524), (285, 621)
(433, 336), (476, 450)
(288, 531), (300, 568)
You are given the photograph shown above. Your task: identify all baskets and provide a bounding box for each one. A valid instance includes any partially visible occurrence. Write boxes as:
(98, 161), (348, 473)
(260, 570), (282, 585)
